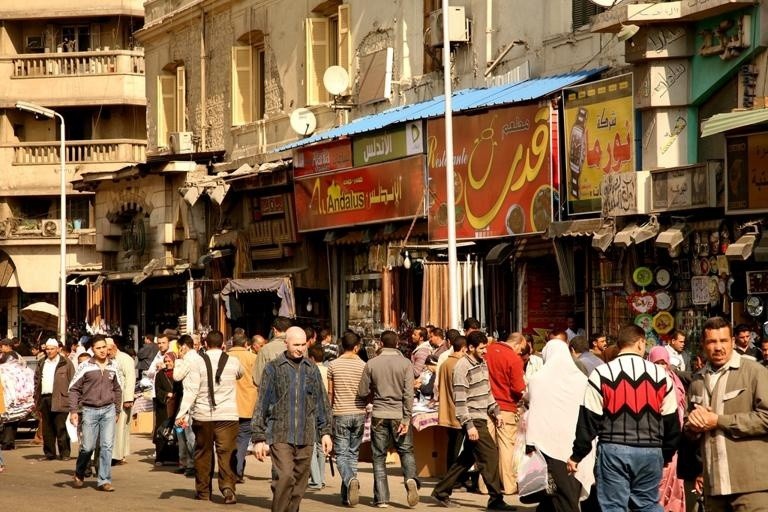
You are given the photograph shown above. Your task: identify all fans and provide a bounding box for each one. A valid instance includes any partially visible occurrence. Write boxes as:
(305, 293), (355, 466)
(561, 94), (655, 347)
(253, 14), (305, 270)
(743, 294), (764, 325)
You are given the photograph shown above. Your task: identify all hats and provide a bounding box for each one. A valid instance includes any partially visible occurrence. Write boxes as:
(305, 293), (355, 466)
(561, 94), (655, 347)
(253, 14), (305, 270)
(45, 338), (58, 347)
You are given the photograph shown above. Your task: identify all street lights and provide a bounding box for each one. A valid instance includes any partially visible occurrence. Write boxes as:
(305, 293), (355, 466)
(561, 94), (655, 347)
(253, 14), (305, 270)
(12, 99), (72, 349)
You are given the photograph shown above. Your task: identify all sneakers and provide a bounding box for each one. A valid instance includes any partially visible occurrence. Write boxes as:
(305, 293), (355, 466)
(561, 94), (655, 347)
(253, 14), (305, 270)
(38, 455), (127, 491)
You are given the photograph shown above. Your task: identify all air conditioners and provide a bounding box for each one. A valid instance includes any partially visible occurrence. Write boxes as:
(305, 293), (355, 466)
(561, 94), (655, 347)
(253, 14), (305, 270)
(428, 4), (471, 49)
(40, 218), (62, 237)
(168, 131), (194, 155)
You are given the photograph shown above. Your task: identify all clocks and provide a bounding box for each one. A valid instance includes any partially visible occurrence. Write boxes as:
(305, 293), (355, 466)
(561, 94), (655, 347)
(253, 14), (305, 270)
(632, 255), (732, 352)
(746, 271), (767, 294)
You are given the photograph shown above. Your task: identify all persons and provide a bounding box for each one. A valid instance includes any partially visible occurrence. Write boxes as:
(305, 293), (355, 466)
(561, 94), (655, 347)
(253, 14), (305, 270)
(2, 318), (768, 512)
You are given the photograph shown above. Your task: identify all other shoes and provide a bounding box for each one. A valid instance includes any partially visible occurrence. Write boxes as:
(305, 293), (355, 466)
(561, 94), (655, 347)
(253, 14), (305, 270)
(487, 501), (515, 511)
(322, 483), (326, 489)
(432, 492), (461, 509)
(370, 502), (389, 507)
(472, 486), (481, 494)
(195, 493), (211, 500)
(236, 476), (245, 483)
(307, 487), (320, 491)
(341, 499), (350, 507)
(223, 488), (236, 503)
(175, 468), (185, 474)
(348, 479), (359, 506)
(184, 470), (195, 476)
(408, 479), (419, 507)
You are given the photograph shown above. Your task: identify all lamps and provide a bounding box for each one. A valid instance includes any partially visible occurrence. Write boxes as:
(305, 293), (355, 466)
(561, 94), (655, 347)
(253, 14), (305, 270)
(402, 250), (412, 269)
(197, 254), (211, 267)
(272, 301), (277, 316)
(323, 230), (348, 243)
(91, 275), (105, 292)
(723, 235), (757, 262)
(305, 296), (313, 313)
(484, 242), (513, 268)
(382, 223), (396, 236)
(591, 212), (691, 252)
(360, 228), (375, 244)
(131, 256), (188, 285)
(615, 0), (640, 47)
